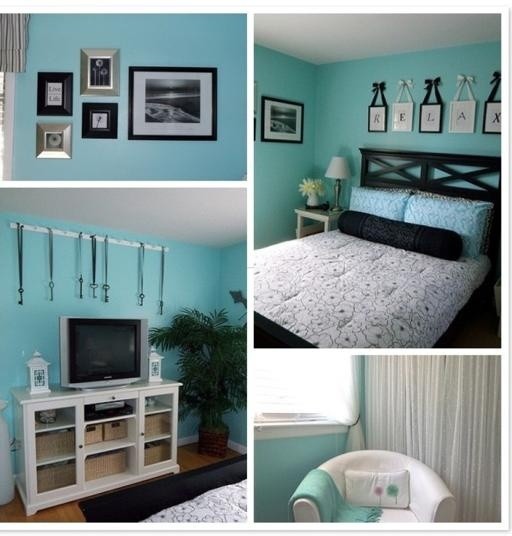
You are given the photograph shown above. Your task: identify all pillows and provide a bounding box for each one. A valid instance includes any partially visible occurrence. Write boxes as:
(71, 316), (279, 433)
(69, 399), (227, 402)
(403, 189), (494, 263)
(349, 185), (412, 223)
(342, 471), (411, 510)
(338, 210), (463, 261)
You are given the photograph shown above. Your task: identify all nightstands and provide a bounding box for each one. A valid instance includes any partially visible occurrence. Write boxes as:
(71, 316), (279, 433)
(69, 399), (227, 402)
(293, 205), (347, 239)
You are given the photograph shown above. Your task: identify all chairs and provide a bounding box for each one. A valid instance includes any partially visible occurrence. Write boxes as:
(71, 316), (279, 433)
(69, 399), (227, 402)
(286, 449), (458, 522)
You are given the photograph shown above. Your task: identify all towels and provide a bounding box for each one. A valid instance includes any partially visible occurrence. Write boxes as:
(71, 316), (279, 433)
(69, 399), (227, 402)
(287, 469), (384, 523)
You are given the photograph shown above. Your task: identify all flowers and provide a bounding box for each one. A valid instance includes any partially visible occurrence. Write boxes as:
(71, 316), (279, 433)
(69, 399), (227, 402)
(299, 177), (326, 197)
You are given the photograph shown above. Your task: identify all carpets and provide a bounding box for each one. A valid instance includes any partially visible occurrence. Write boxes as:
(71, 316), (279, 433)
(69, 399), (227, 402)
(78, 453), (247, 522)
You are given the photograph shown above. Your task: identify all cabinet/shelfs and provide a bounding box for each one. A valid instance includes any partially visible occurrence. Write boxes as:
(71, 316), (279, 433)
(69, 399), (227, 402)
(8, 378), (184, 517)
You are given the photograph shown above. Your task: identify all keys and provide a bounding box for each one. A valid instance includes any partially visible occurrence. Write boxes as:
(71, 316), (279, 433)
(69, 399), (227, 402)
(18, 288), (24, 305)
(79, 279), (83, 299)
(49, 281), (54, 301)
(139, 294), (145, 306)
(102, 285), (110, 302)
(90, 284), (98, 299)
(160, 301), (163, 315)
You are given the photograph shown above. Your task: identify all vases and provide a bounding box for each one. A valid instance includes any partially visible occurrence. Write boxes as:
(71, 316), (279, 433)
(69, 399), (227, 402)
(307, 192), (321, 206)
(367, 100), (501, 134)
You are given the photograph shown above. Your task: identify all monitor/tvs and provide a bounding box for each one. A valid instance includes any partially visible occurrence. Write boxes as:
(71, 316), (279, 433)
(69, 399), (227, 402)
(60, 316), (148, 392)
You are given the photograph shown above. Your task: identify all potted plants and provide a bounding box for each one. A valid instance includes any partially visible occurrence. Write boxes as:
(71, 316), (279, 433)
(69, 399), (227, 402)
(146, 305), (247, 458)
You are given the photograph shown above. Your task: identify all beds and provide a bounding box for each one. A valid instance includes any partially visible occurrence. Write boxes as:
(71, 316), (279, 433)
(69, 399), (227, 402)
(254, 147), (501, 348)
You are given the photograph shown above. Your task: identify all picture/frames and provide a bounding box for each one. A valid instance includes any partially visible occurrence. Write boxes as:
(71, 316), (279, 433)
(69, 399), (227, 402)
(80, 48), (121, 97)
(128, 66), (218, 142)
(81, 102), (118, 140)
(35, 123), (72, 159)
(261, 95), (304, 144)
(37, 71), (73, 116)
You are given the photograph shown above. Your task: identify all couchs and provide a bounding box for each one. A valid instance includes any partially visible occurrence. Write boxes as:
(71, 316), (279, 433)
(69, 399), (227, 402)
(140, 478), (247, 522)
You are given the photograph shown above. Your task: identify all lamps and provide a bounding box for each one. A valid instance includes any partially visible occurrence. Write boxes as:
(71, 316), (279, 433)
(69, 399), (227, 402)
(324, 156), (351, 212)
